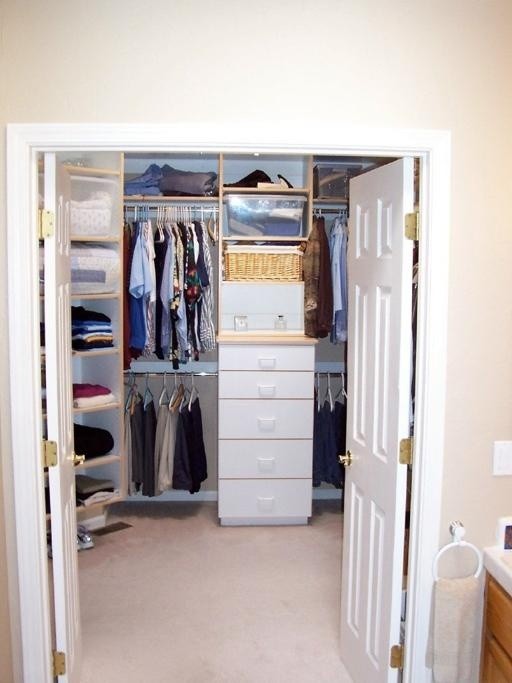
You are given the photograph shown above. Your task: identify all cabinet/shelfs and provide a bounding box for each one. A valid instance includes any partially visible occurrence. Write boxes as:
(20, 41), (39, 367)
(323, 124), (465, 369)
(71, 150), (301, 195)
(478, 571), (512, 683)
(221, 154), (313, 339)
(39, 152), (123, 535)
(311, 161), (348, 500)
(218, 339), (318, 526)
(219, 284), (305, 335)
(124, 152), (220, 501)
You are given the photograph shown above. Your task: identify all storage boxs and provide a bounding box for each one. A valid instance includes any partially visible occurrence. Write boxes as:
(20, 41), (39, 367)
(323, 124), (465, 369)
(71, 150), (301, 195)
(67, 175), (117, 238)
(313, 164), (363, 198)
(223, 194), (308, 239)
(70, 244), (119, 295)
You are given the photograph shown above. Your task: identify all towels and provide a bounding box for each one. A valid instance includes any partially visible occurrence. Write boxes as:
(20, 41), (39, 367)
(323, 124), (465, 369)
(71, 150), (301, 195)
(425, 574), (483, 681)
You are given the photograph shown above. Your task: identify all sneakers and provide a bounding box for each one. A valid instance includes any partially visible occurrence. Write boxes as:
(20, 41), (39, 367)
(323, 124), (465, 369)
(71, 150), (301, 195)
(77, 533), (95, 550)
